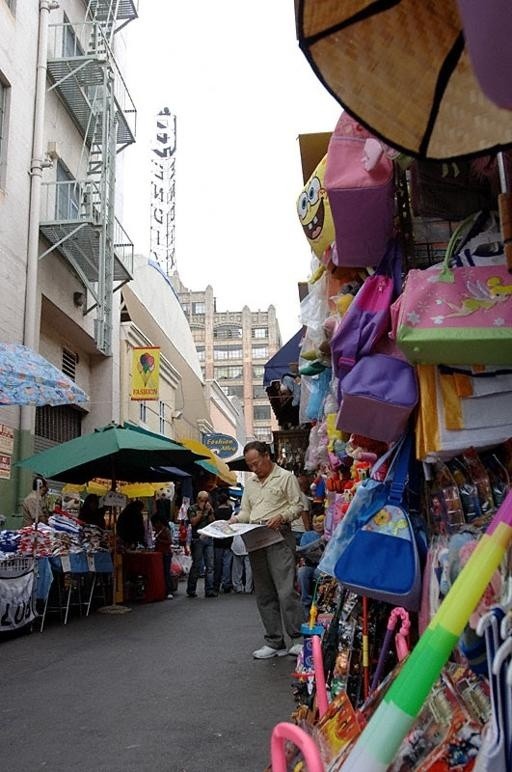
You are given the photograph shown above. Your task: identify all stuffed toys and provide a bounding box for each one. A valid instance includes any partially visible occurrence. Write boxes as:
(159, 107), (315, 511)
(296, 155), (379, 541)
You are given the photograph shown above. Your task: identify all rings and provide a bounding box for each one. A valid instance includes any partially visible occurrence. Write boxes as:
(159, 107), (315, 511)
(278, 525), (282, 528)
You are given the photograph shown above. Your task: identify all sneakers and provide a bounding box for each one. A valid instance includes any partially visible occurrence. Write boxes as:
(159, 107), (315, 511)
(251, 645), (290, 660)
(186, 587), (254, 598)
(289, 642), (305, 657)
(168, 592), (174, 599)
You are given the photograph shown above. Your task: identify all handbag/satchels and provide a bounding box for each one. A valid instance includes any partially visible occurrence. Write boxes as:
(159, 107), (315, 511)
(316, 435), (427, 614)
(322, 108), (396, 267)
(388, 266), (512, 367)
(328, 270), (420, 443)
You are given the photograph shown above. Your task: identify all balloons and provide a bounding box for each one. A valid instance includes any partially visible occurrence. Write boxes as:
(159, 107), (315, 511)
(137, 352), (155, 387)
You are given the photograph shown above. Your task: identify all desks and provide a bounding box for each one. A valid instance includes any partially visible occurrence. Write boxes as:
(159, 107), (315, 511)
(0, 540), (173, 644)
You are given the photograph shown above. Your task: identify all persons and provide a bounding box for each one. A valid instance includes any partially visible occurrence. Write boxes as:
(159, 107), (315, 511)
(151, 512), (176, 599)
(297, 508), (328, 622)
(79, 493), (110, 529)
(168, 490), (256, 599)
(227, 441), (303, 659)
(21, 477), (51, 527)
(116, 500), (148, 547)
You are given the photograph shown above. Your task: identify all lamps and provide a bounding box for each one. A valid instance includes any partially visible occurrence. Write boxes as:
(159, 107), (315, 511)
(73, 290), (87, 309)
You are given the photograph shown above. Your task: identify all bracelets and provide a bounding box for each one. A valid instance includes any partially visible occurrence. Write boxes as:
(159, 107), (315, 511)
(280, 513), (286, 524)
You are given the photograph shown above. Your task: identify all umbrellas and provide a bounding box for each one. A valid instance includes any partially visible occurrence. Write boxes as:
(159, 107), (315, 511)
(11, 419), (212, 615)
